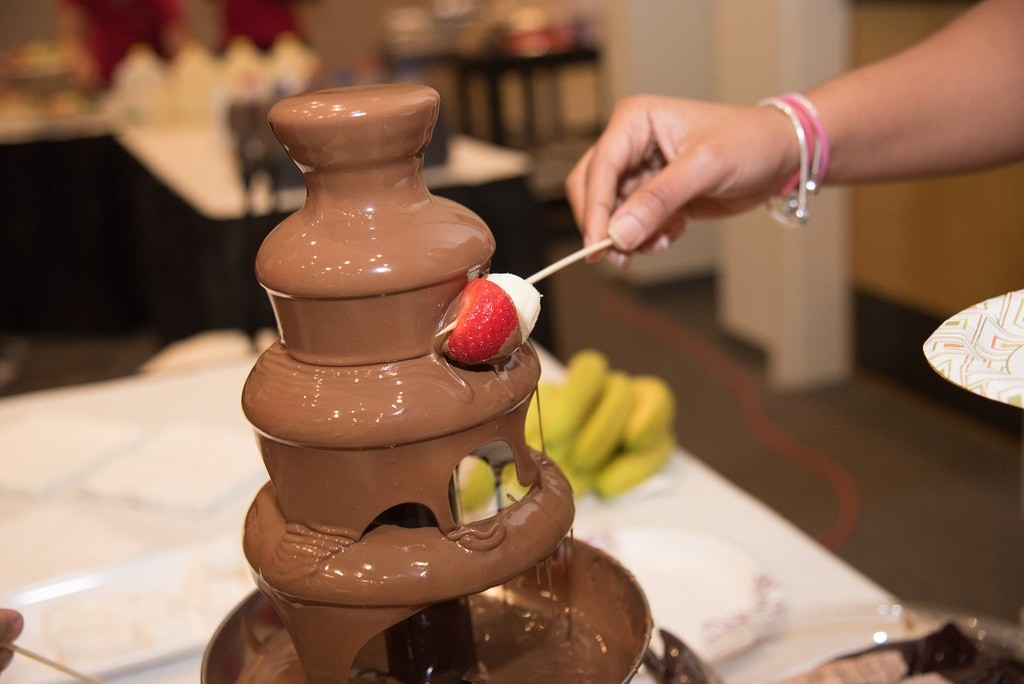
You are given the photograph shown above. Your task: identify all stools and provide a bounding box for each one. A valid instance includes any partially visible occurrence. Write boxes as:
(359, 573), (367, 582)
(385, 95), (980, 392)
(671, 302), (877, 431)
(456, 44), (599, 153)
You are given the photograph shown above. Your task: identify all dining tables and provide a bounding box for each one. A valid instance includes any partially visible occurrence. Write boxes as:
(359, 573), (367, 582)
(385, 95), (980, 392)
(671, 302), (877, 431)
(2, 341), (922, 681)
(114, 105), (550, 352)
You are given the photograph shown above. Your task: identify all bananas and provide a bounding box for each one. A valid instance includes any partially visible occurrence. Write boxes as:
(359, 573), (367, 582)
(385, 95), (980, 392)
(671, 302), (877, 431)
(460, 348), (674, 518)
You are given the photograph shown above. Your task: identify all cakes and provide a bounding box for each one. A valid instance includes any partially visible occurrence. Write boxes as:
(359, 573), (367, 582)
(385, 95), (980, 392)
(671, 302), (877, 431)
(5, 0), (580, 684)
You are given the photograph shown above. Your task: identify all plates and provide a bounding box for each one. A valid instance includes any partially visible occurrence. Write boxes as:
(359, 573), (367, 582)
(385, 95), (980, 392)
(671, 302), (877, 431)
(922, 283), (1024, 416)
(594, 518), (789, 662)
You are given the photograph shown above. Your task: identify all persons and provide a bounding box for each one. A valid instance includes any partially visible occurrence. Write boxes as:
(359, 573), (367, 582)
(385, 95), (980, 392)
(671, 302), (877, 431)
(562, 0), (1024, 266)
(0, 610), (23, 674)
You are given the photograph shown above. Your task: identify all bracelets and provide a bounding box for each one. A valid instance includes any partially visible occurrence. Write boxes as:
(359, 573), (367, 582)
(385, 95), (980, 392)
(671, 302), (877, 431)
(754, 99), (829, 226)
